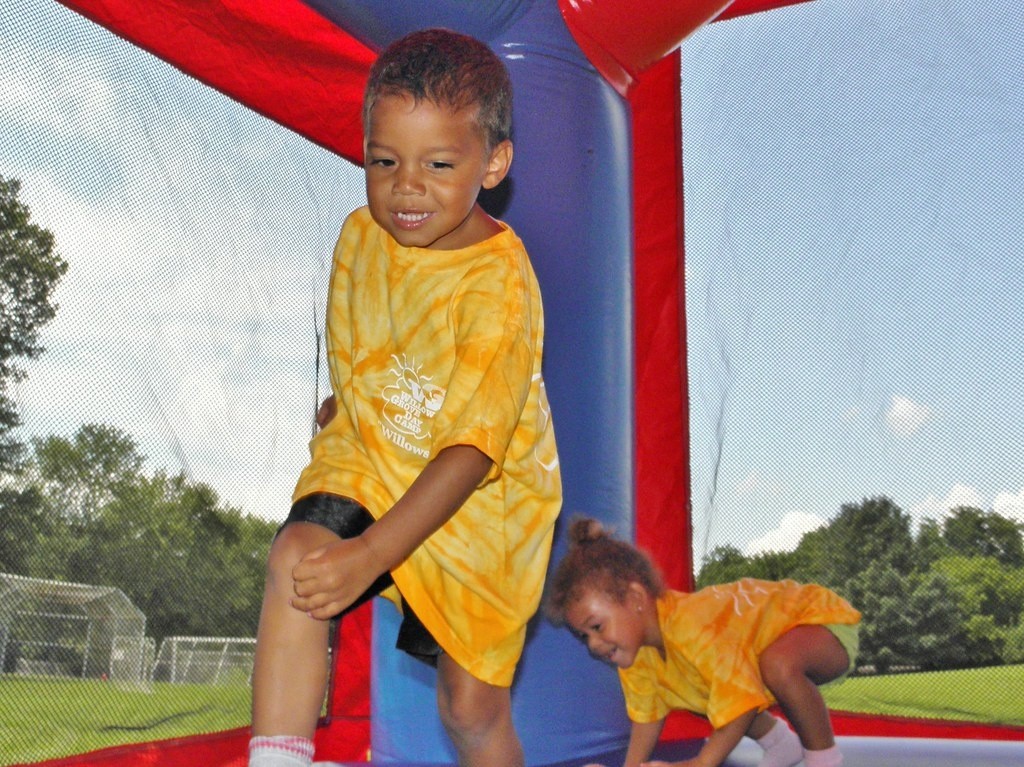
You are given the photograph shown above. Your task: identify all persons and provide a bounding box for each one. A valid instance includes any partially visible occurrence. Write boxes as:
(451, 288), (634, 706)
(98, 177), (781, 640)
(249, 28), (563, 767)
(544, 520), (862, 767)
